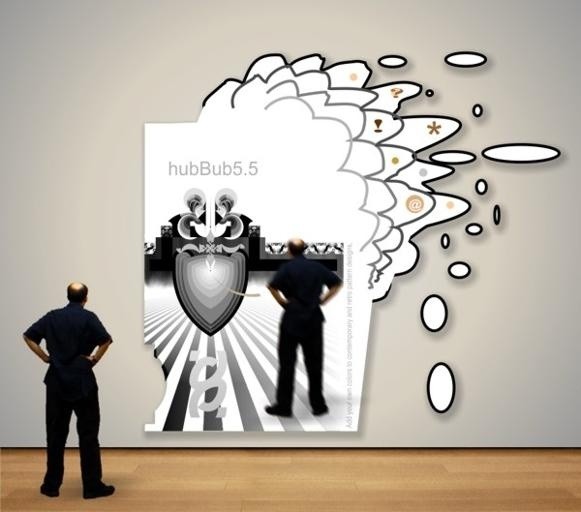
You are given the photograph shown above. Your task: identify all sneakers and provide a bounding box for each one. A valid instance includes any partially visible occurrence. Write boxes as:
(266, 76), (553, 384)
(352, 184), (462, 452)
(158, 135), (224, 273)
(313, 406), (328, 415)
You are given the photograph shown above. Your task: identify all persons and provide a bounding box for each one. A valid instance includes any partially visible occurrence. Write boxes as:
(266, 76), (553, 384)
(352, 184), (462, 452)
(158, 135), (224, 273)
(266, 238), (342, 418)
(22, 282), (116, 499)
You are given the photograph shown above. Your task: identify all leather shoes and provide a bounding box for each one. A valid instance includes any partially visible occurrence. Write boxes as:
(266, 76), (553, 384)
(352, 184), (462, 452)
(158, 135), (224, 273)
(266, 405), (291, 416)
(40, 485), (59, 497)
(83, 486), (114, 499)
